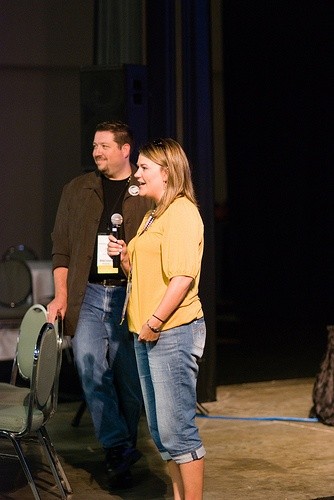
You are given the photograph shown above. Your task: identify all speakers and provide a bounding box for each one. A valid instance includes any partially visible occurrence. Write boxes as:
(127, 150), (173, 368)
(77, 61), (147, 173)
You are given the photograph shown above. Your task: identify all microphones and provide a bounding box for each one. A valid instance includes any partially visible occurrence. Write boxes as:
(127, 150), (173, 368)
(110, 213), (124, 268)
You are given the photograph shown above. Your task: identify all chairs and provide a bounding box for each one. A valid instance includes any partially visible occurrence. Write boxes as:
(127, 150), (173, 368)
(0, 244), (74, 500)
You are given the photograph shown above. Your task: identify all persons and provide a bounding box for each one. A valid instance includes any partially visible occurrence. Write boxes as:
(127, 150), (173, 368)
(108, 138), (206, 500)
(46, 122), (156, 491)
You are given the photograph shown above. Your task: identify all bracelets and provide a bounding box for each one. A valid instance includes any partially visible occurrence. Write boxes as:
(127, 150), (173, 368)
(146, 320), (160, 333)
(152, 314), (165, 323)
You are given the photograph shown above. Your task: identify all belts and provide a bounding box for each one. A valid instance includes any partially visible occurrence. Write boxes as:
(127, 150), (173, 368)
(88, 276), (126, 287)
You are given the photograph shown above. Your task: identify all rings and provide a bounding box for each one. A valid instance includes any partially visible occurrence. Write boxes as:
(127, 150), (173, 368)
(47, 311), (49, 314)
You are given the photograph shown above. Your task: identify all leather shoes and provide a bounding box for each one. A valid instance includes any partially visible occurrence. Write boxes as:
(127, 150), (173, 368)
(101, 447), (143, 491)
